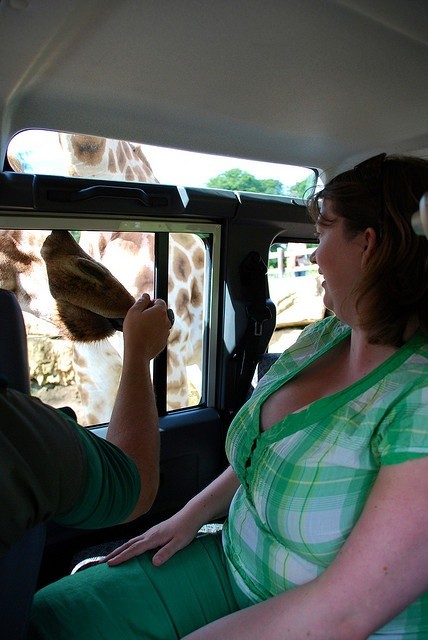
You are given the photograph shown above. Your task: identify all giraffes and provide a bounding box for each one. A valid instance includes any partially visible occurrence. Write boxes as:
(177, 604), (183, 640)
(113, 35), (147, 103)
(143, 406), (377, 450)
(0, 133), (207, 426)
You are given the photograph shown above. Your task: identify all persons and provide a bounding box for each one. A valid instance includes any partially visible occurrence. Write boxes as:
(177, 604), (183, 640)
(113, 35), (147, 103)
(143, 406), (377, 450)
(0, 293), (176, 640)
(32, 155), (428, 640)
